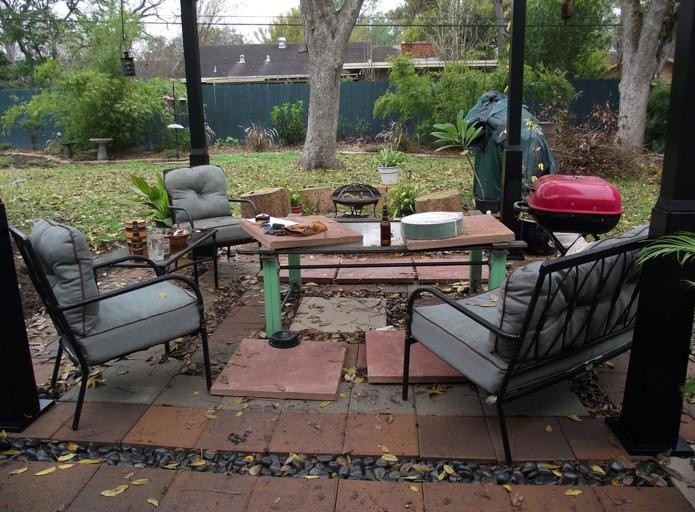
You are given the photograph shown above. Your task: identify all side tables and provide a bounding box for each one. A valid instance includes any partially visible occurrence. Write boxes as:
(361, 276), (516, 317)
(88, 225), (221, 333)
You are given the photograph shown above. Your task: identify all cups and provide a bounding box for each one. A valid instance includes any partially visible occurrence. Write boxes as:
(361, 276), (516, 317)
(149, 233), (165, 262)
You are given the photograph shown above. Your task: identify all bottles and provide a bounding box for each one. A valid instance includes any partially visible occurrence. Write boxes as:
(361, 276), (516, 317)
(131, 221), (144, 262)
(380, 205), (391, 247)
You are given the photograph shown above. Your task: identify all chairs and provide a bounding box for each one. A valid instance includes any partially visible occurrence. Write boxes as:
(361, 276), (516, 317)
(6, 213), (215, 430)
(330, 180), (380, 223)
(159, 162), (266, 285)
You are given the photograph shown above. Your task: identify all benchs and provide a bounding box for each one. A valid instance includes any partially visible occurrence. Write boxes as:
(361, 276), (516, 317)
(403, 225), (652, 462)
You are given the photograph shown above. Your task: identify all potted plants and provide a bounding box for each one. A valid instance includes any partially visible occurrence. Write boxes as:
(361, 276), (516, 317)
(291, 191), (304, 212)
(372, 146), (406, 184)
